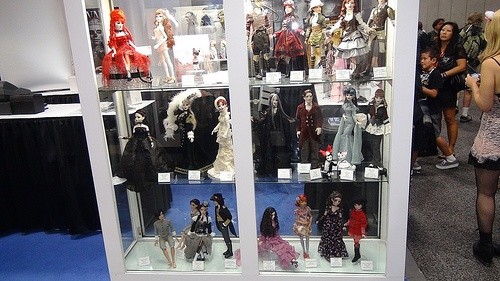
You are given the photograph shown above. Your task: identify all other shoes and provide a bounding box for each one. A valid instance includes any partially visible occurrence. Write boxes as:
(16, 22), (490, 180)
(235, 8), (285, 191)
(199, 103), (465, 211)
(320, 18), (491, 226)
(303, 252), (310, 259)
(377, 165), (383, 171)
(363, 67), (373, 75)
(349, 64), (362, 79)
(255, 74), (262, 79)
(168, 78), (177, 84)
(127, 77), (132, 81)
(368, 163), (374, 167)
(473, 233), (495, 267)
(197, 254), (201, 261)
(163, 78), (170, 82)
(201, 256), (205, 261)
(461, 114), (473, 122)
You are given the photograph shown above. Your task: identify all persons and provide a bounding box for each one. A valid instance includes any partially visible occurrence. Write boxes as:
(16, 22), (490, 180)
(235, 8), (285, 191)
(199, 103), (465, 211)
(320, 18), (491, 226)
(85, 1), (394, 270)
(455, 12), (486, 123)
(465, 9), (500, 266)
(410, 17), (467, 178)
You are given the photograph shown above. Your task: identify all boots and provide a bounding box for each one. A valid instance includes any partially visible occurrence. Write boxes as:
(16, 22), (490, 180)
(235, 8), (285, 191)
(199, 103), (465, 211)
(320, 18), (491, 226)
(223, 248), (228, 255)
(225, 243), (233, 258)
(351, 244), (361, 264)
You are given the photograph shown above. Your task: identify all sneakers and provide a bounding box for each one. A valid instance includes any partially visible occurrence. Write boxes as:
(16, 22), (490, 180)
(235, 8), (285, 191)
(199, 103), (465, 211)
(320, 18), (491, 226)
(413, 162), (422, 173)
(439, 151), (456, 158)
(436, 159), (459, 169)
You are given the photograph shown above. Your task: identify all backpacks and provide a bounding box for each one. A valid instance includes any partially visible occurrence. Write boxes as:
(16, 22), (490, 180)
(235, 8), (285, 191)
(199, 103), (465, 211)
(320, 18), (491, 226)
(463, 29), (482, 62)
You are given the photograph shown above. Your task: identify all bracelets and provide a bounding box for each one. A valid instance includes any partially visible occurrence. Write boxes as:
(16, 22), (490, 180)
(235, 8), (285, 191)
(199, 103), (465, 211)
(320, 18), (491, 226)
(442, 72), (447, 78)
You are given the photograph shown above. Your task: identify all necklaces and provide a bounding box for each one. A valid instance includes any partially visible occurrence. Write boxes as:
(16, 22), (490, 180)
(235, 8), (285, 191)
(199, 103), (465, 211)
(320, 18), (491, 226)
(440, 52), (445, 57)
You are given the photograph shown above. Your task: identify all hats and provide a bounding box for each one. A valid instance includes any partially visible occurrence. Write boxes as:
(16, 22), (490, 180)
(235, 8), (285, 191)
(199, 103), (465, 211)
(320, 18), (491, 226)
(306, 0), (325, 13)
(210, 193), (226, 201)
(484, 11), (495, 22)
(214, 96), (227, 110)
(283, 0), (296, 10)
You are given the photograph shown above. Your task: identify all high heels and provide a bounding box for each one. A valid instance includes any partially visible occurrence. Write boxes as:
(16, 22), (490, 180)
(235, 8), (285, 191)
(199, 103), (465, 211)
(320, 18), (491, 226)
(291, 259), (298, 265)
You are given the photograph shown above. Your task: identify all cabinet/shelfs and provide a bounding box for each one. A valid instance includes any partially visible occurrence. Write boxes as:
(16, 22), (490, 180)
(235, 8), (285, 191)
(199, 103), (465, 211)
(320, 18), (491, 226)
(64, 0), (420, 281)
